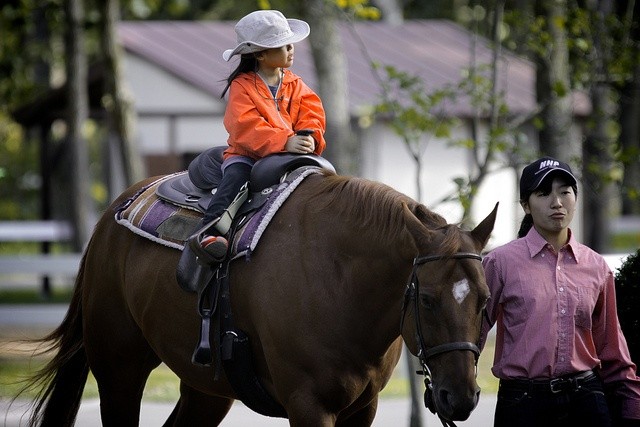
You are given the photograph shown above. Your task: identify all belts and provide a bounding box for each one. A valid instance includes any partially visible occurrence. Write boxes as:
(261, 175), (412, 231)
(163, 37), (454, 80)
(500, 370), (594, 394)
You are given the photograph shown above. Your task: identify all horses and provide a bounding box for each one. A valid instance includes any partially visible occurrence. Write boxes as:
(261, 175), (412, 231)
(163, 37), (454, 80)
(0, 165), (500, 427)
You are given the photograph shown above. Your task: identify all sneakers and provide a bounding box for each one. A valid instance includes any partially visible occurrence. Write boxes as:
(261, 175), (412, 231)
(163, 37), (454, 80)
(200, 231), (229, 259)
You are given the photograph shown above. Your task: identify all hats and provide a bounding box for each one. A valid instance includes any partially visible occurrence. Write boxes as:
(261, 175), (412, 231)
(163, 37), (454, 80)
(519, 157), (577, 193)
(222, 9), (311, 62)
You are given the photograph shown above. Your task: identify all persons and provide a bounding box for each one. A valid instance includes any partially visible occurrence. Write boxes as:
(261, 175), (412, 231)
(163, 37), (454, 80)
(478, 158), (639, 427)
(198, 11), (326, 259)
